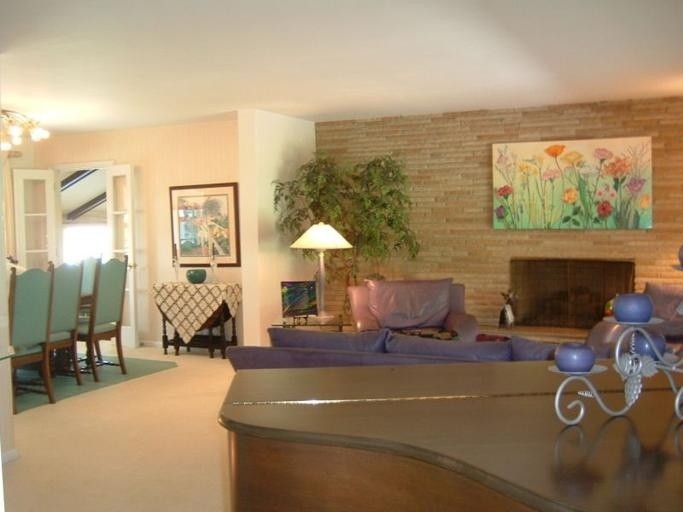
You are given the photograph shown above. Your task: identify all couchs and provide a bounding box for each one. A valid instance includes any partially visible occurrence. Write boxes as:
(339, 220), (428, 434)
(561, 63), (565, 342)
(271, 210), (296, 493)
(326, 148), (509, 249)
(227, 325), (557, 371)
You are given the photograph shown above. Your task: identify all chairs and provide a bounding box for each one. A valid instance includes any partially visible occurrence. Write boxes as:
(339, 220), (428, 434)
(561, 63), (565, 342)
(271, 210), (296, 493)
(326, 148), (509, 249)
(643, 282), (682, 363)
(347, 275), (478, 345)
(3, 252), (129, 404)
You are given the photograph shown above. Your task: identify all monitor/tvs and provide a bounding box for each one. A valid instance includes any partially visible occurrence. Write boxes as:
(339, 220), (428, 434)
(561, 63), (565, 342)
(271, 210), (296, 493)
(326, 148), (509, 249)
(508, 256), (635, 330)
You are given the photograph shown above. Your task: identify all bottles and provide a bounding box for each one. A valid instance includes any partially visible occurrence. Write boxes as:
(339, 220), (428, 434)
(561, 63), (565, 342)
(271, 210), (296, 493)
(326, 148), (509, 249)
(604, 293), (619, 316)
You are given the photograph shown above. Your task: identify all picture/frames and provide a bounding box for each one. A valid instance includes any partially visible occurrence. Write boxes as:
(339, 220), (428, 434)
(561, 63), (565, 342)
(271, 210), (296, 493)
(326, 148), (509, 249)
(169, 181), (241, 268)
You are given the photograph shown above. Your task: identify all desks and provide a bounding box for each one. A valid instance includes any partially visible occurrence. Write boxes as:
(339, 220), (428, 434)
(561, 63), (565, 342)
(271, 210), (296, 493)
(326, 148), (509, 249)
(585, 319), (665, 360)
(272, 318), (355, 333)
(151, 281), (240, 359)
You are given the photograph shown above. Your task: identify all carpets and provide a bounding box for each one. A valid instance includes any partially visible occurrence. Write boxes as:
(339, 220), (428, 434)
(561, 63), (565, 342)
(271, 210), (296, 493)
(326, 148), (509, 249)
(13, 355), (179, 412)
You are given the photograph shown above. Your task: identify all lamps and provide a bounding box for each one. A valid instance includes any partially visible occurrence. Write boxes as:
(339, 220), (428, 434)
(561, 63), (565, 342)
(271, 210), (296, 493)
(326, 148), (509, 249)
(287, 221), (354, 321)
(0, 107), (50, 156)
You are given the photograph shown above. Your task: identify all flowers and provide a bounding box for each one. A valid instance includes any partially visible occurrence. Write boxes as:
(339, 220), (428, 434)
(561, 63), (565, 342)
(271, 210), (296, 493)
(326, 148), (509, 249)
(493, 142), (652, 230)
